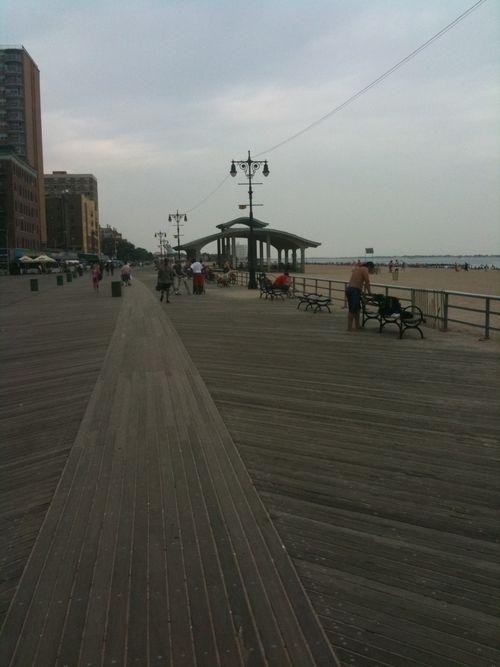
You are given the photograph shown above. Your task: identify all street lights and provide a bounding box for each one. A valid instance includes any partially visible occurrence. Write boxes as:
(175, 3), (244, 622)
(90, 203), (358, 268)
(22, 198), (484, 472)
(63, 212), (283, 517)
(155, 229), (167, 263)
(168, 210), (187, 261)
(230, 150), (270, 290)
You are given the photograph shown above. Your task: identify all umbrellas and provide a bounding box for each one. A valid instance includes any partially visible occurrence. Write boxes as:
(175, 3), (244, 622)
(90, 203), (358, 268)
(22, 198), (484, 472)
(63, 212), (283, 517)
(14, 254), (57, 263)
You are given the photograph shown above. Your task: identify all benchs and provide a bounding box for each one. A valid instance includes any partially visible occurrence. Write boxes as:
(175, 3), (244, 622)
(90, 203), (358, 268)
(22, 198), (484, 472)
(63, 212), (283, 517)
(361, 293), (425, 340)
(259, 287), (331, 313)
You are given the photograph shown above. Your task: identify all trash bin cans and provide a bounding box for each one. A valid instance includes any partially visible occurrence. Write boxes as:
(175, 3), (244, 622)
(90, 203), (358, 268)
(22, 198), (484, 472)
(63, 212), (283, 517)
(392, 271), (398, 280)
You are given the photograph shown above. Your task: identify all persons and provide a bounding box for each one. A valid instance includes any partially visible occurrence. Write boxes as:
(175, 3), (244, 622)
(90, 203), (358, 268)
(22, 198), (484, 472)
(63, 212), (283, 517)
(155, 258), (175, 303)
(388, 259), (405, 272)
(272, 271), (292, 293)
(435, 263), (495, 271)
(346, 262), (374, 332)
(172, 259), (233, 295)
(89, 259), (132, 291)
